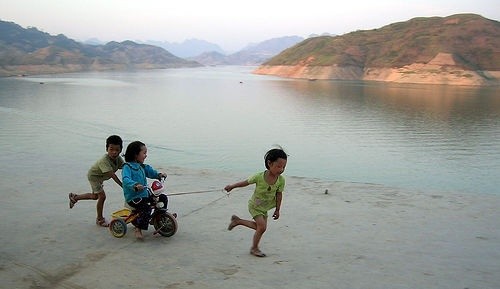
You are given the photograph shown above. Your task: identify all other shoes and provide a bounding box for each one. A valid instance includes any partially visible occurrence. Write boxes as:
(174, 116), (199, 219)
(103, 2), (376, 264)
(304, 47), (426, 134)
(250, 247), (265, 257)
(227, 215), (240, 230)
(135, 230), (144, 240)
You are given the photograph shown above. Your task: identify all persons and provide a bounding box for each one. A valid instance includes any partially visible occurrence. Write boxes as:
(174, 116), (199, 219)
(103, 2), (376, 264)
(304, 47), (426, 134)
(122, 141), (167, 239)
(69, 135), (125, 227)
(224, 149), (287, 257)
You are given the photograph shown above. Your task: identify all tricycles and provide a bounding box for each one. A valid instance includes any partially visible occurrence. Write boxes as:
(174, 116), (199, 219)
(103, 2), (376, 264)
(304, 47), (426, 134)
(109, 174), (179, 240)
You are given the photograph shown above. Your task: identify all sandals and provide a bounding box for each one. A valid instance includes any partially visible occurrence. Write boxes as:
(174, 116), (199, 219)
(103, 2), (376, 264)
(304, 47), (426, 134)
(96, 217), (107, 227)
(69, 193), (77, 208)
